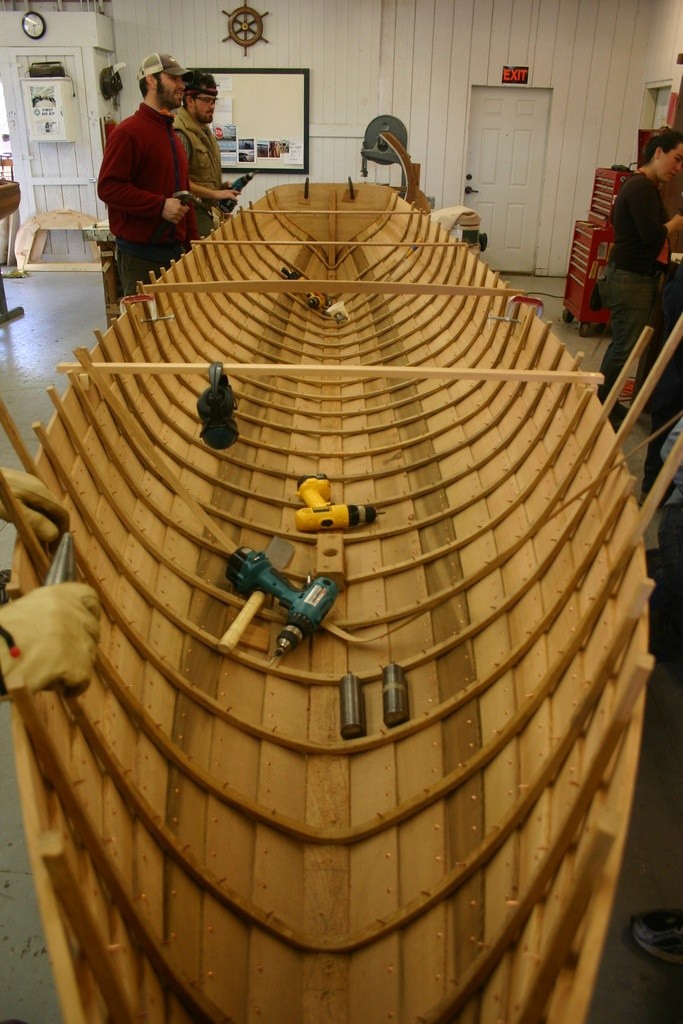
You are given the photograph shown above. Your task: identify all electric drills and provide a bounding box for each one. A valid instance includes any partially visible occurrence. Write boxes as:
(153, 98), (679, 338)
(225, 542), (339, 670)
(283, 266), (327, 308)
(218, 168), (263, 213)
(294, 472), (387, 533)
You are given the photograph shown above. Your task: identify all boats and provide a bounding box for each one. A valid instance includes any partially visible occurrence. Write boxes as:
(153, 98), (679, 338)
(0, 129), (683, 1023)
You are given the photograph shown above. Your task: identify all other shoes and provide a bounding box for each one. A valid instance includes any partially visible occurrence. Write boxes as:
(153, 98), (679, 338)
(609, 399), (629, 425)
(638, 489), (647, 506)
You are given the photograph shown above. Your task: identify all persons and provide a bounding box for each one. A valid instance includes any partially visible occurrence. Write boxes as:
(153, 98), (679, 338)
(647, 419), (683, 664)
(173, 72), (241, 239)
(97, 55), (200, 297)
(632, 908), (683, 965)
(638, 259), (683, 505)
(0, 466), (102, 698)
(598, 132), (683, 428)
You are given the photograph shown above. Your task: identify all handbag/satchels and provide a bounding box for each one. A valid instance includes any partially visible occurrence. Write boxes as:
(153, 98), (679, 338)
(590, 282), (601, 312)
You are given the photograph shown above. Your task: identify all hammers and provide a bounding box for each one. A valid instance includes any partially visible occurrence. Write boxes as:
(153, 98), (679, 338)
(150, 189), (203, 245)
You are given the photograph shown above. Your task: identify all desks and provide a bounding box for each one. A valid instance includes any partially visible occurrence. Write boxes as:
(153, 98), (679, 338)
(0, 179), (25, 323)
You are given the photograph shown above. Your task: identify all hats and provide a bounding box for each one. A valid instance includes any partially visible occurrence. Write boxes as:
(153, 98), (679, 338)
(137, 52), (194, 83)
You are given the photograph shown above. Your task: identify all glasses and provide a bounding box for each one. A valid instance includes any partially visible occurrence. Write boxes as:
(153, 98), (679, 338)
(192, 96), (218, 104)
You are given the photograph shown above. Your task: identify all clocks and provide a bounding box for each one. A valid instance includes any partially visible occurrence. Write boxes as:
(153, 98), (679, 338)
(22, 11), (46, 39)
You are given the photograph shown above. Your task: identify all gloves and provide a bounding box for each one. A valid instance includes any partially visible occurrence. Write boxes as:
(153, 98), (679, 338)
(0, 465), (70, 554)
(0, 582), (102, 698)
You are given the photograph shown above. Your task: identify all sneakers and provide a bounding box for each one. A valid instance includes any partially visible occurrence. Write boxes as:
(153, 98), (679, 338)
(630, 908), (683, 965)
